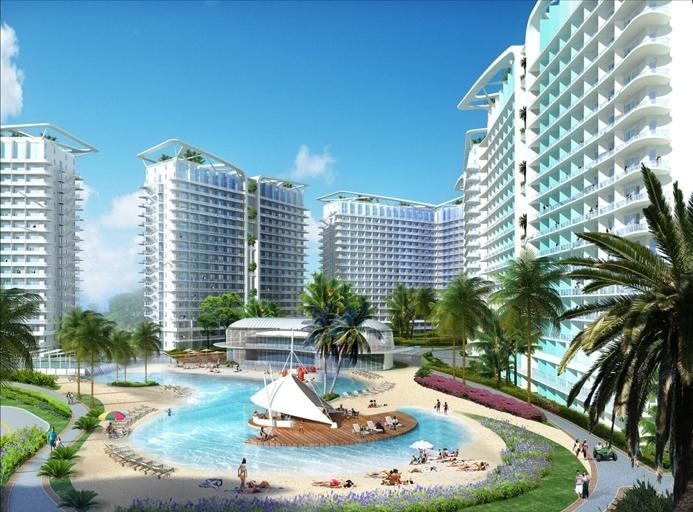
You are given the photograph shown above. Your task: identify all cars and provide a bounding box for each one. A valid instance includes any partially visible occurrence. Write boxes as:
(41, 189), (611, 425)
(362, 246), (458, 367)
(593, 445), (617, 462)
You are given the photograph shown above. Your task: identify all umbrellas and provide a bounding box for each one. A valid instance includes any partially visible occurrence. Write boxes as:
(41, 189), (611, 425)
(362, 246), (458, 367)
(409, 440), (433, 450)
(97, 410), (125, 421)
(183, 348), (225, 362)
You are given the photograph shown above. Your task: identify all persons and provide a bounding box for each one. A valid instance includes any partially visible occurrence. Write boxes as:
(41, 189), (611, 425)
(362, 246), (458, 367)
(572, 439), (580, 459)
(264, 408), (268, 418)
(581, 471), (590, 498)
(581, 439), (588, 458)
(298, 419), (304, 431)
(367, 399), (373, 408)
(373, 399), (377, 408)
(573, 470), (583, 500)
(441, 401), (448, 415)
(373, 419), (384, 434)
(66, 392), (70, 404)
(245, 480), (269, 489)
(105, 423), (120, 440)
(336, 404), (344, 410)
(238, 458), (247, 493)
(391, 416), (398, 430)
(259, 426), (268, 441)
(70, 392), (73, 403)
(311, 479), (352, 488)
(253, 410), (262, 418)
(381, 449), (488, 486)
(434, 398), (440, 413)
(594, 441), (602, 450)
(47, 427), (57, 452)
(602, 441), (608, 448)
(346, 408), (355, 419)
(56, 437), (63, 449)
